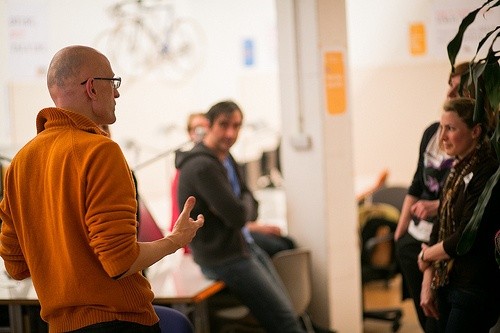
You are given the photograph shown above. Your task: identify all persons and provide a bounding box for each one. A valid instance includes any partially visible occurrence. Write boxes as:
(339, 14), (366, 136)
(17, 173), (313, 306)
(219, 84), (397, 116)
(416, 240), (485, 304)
(394, 62), (477, 333)
(170, 114), (210, 254)
(176, 101), (303, 333)
(419, 98), (500, 333)
(1, 46), (203, 333)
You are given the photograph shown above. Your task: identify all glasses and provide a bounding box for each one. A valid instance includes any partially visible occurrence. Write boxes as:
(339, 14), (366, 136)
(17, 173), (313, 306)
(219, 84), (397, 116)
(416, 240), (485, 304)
(79, 74), (124, 90)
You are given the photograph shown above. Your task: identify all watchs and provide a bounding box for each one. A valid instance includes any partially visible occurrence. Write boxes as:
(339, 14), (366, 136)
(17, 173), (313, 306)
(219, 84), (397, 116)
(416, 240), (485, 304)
(419, 249), (427, 263)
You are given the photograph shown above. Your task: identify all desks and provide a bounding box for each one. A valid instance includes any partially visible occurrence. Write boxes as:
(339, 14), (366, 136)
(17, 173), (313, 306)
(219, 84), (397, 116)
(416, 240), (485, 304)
(0, 245), (227, 333)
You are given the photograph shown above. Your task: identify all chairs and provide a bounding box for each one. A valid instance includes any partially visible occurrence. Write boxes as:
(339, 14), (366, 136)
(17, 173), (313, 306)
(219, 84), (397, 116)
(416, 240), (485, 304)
(213, 246), (316, 333)
